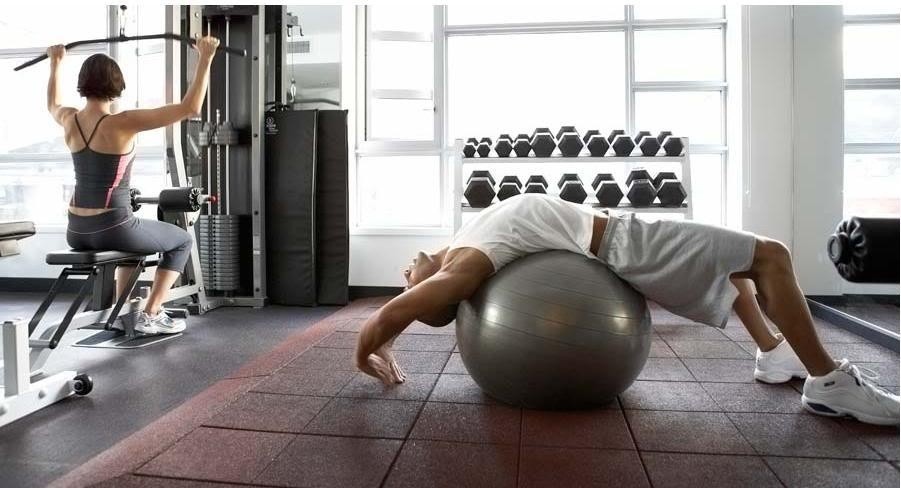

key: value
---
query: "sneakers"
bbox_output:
[132,306,187,335]
[109,315,126,332]
[752,332,811,384]
[800,357,899,429]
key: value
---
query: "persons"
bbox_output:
[45,35,220,336]
[357,192,900,432]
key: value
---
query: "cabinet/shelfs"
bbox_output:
[452,135,694,238]
